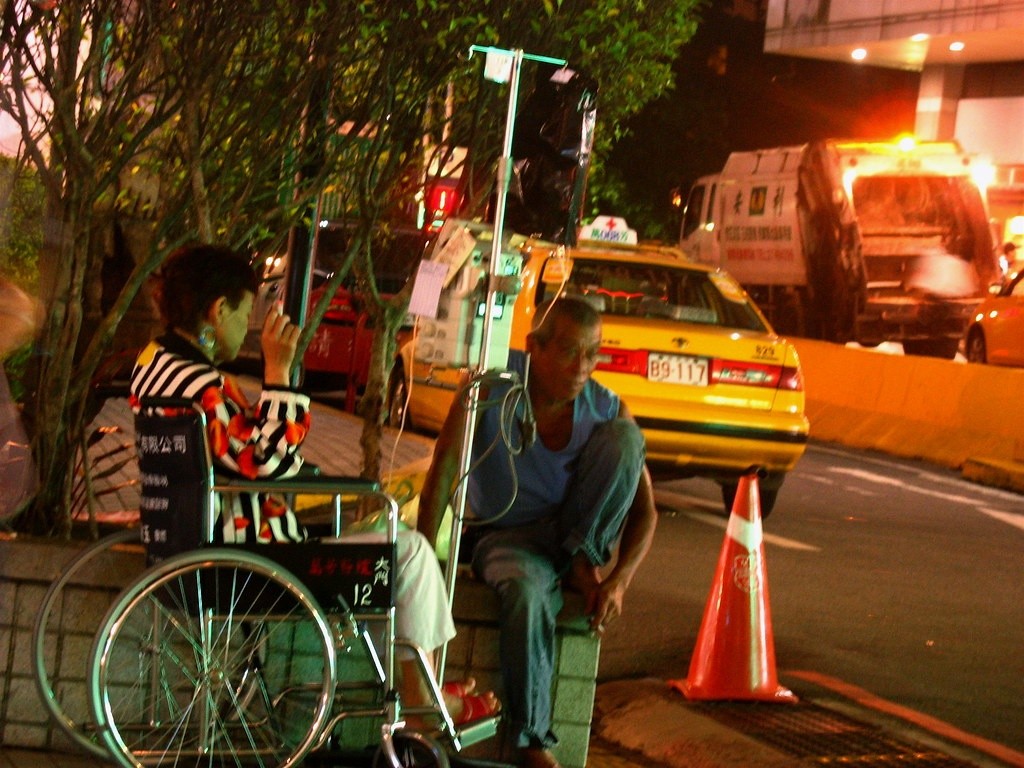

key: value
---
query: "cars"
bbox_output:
[257,253,404,387]
[961,261,1024,366]
[392,216,808,518]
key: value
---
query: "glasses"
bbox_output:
[538,340,595,362]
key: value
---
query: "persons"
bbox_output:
[416,297,658,768]
[130,244,502,739]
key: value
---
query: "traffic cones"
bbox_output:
[669,471,798,704]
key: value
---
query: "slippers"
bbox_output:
[398,677,500,729]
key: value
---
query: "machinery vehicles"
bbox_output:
[686,137,1005,355]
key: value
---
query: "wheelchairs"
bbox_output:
[30,383,505,768]
